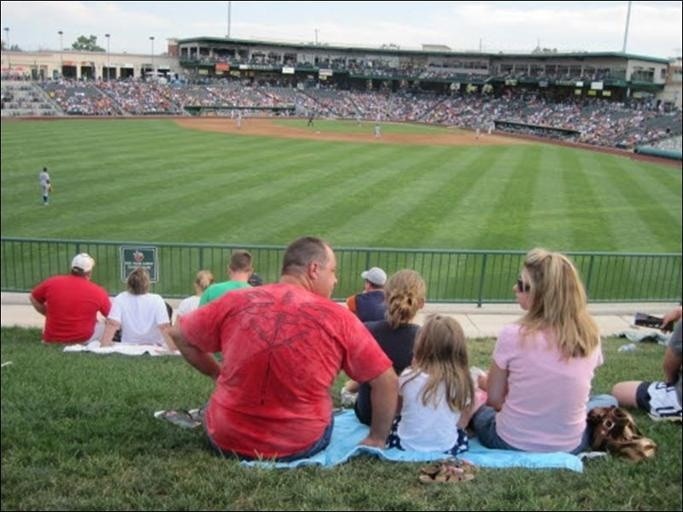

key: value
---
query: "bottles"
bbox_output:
[361,266,387,287]
[71,252,97,275]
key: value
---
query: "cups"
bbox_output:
[517,279,531,294]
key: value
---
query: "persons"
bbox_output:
[30,253,113,346]
[1,52,682,148]
[100,268,177,351]
[612,305,683,420]
[247,274,263,286]
[168,236,399,462]
[386,312,478,455]
[473,248,618,455]
[346,267,390,324]
[171,270,215,326]
[39,167,51,206]
[345,268,427,427]
[198,250,255,363]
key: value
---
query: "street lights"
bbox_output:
[586,404,657,465]
